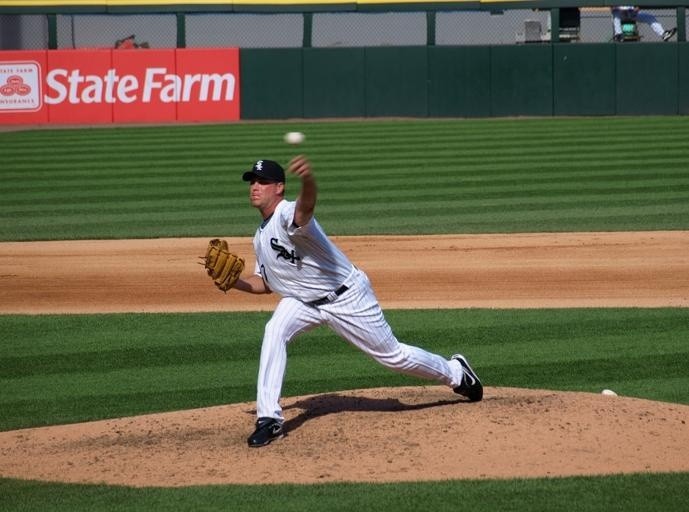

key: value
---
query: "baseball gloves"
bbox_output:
[204,240,245,293]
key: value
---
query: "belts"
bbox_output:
[305,284,348,308]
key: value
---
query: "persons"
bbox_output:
[204,154,483,447]
[611,6,677,42]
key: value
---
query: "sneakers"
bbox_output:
[451,353,485,403]
[247,417,285,447]
[662,27,676,41]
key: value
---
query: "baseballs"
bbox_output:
[286,132,303,144]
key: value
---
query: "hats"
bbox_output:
[241,159,286,184]
